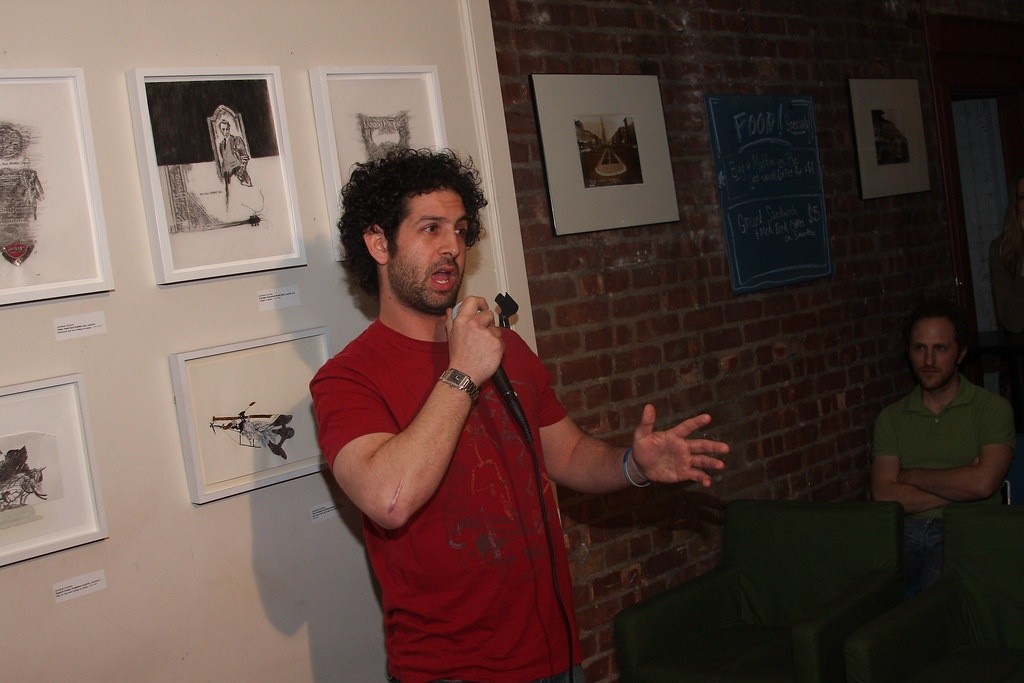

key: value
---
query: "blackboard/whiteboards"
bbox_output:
[703,93,833,294]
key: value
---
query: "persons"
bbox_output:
[989,167,1024,434]
[308,149,730,683]
[871,302,1017,596]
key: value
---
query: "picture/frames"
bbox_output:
[531,72,685,239]
[128,65,308,286]
[0,65,115,306]
[0,372,109,567]
[310,65,448,262]
[845,76,932,202]
[173,325,333,505]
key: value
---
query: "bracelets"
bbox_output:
[623,448,651,487]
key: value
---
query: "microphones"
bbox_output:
[451,301,535,443]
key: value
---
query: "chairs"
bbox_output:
[613,499,1024,683]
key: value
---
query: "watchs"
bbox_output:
[438,368,479,402]
